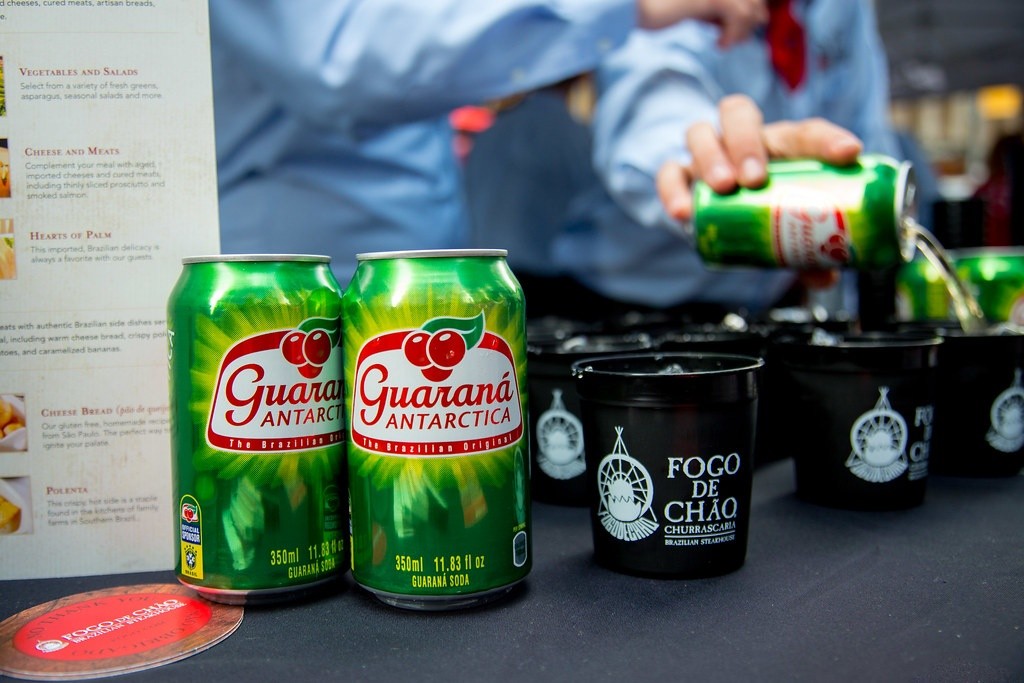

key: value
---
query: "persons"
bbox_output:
[208,0,936,325]
[943,130,1024,248]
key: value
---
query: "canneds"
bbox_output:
[895,246,1024,325]
[689,150,919,268]
[164,248,536,611]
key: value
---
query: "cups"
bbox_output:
[521,304,1024,580]
